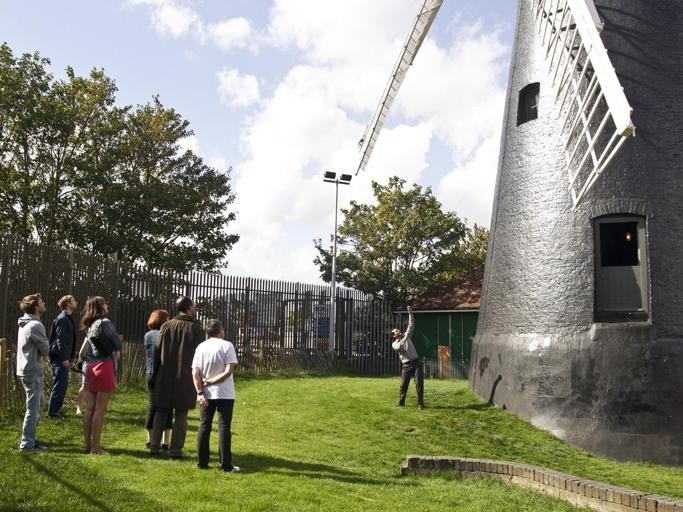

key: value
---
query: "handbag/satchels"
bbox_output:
[88,317,118,358]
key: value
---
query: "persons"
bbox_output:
[390,306,425,409]
[47,295,78,416]
[16,293,50,451]
[191,318,240,472]
[144,310,173,450]
[81,295,123,454]
[76,357,87,416]
[149,297,206,459]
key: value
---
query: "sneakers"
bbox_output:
[144,438,186,459]
[19,439,49,452]
[227,464,241,473]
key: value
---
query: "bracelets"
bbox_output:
[199,392,204,395]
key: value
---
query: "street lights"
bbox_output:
[323,169,352,349]
